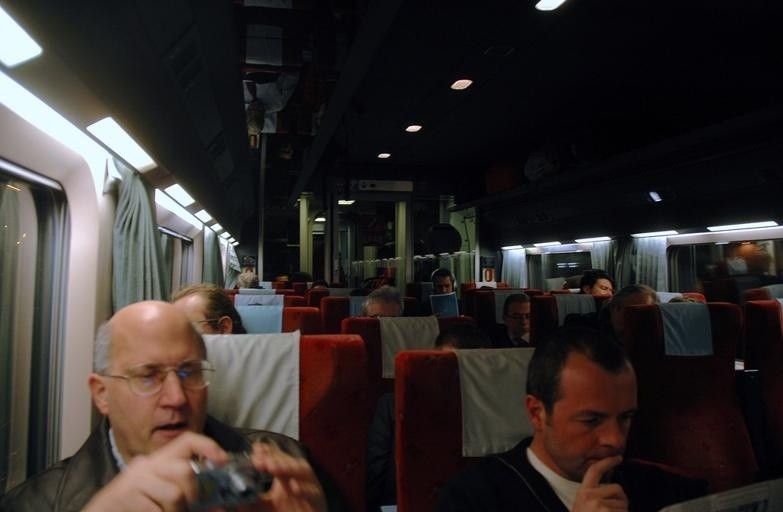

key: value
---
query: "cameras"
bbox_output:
[190,452,273,507]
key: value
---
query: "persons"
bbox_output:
[485,269,660,349]
[362,284,404,319]
[430,268,465,317]
[237,273,264,290]
[434,322,717,512]
[0,300,352,512]
[175,282,244,334]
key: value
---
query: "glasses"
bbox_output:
[502,311,531,323]
[99,356,216,397]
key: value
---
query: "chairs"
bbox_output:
[160,272,781,510]
